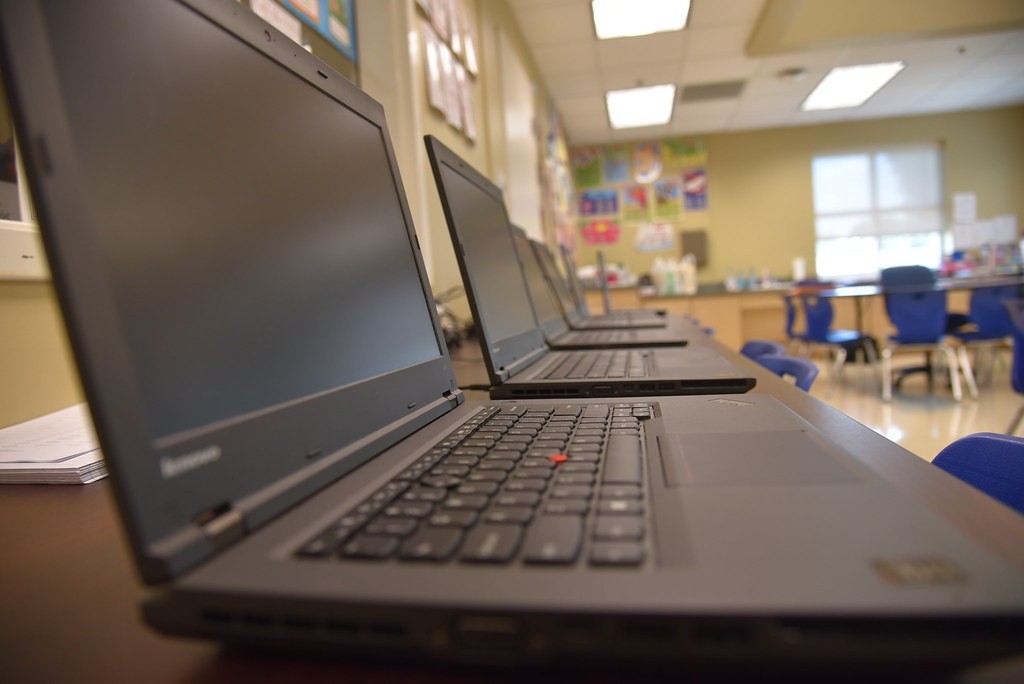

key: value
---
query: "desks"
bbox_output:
[0,275,1024,684]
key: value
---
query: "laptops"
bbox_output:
[0,0,1024,673]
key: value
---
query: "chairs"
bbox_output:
[687,266,1024,516]
